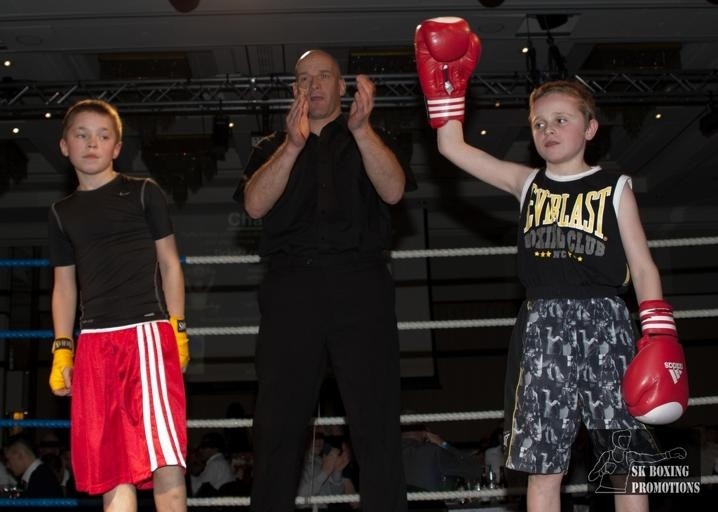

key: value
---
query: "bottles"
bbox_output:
[440,465,497,506]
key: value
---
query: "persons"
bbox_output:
[36,96,193,512]
[416,14,694,512]
[2,418,718,512]
[236,44,418,510]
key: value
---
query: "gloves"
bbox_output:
[167,314,193,371]
[620,299,688,427]
[46,334,76,394]
[413,15,483,129]
